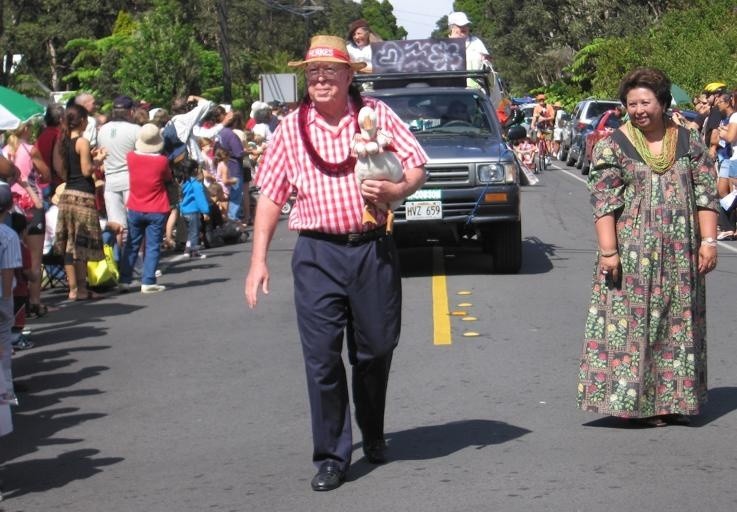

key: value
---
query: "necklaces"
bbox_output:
[465,33,471,48]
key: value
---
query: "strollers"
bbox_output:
[250,187,296,215]
[194,183,254,248]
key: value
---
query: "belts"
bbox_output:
[298,226,387,248]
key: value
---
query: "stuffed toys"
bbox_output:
[349,106,406,237]
[534,109,553,138]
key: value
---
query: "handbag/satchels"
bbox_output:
[87,243,119,284]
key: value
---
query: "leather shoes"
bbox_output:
[310,460,343,491]
[361,431,385,463]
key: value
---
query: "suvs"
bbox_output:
[350,70,525,271]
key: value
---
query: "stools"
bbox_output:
[41,255,69,291]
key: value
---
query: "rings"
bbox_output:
[600,270,610,275]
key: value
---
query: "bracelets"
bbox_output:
[601,249,618,257]
[700,238,717,247]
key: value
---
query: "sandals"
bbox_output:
[716,230,734,240]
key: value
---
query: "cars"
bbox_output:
[515,95,700,186]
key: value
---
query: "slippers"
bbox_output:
[76,292,103,300]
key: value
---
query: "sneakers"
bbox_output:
[141,285,166,294]
[189,253,205,259]
[121,283,129,292]
[11,337,34,351]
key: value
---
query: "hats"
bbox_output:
[552,101,561,108]
[448,11,474,27]
[287,35,367,72]
[135,122,164,153]
[346,20,367,41]
[113,96,133,109]
[537,95,545,100]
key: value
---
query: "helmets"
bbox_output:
[703,81,727,95]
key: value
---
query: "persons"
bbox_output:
[613,104,626,126]
[448,11,493,98]
[576,65,721,426]
[672,82,736,240]
[531,93,555,166]
[445,100,467,126]
[245,35,430,490]
[1,85,295,314]
[0,222,22,435]
[347,18,372,75]
